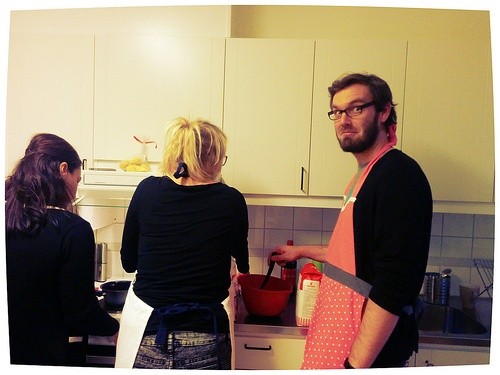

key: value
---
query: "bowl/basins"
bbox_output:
[237,274,293,316]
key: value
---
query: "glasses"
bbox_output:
[219,155,228,166]
[327,101,376,121]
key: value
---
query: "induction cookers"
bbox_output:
[88,298,122,344]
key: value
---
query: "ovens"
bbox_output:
[86,344,116,368]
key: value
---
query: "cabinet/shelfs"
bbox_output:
[5,4,232,171]
[234,335,307,370]
[308,39,495,215]
[220,37,316,207]
[415,342,490,368]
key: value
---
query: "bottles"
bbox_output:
[280,240,298,298]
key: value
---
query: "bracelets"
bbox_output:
[343,358,355,369]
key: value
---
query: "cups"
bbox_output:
[459,284,480,309]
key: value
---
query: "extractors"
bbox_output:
[78,169,161,186]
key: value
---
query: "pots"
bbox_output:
[94,281,132,311]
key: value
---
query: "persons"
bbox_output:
[5,133,120,368]
[120,117,250,370]
[267,73,434,368]
